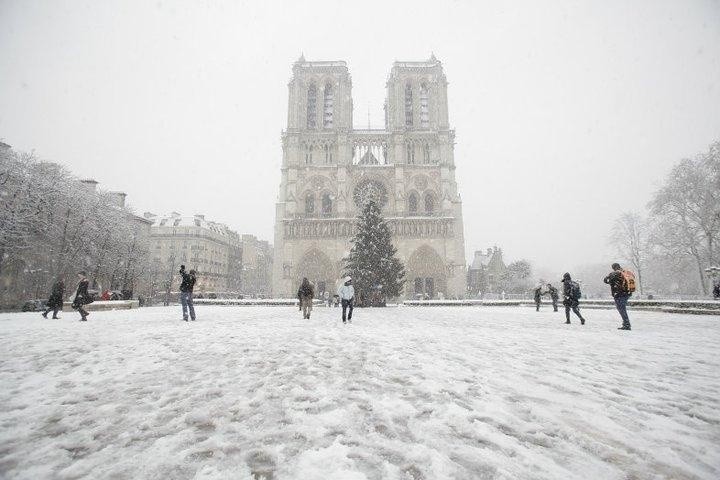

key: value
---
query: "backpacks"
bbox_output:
[621,270,636,292]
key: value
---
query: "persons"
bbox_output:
[561,272,586,325]
[603,262,636,330]
[42,277,65,319]
[297,277,315,319]
[534,284,559,312]
[71,270,94,321]
[179,264,197,321]
[337,276,355,324]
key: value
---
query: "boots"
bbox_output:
[42,313,60,319]
[80,310,89,321]
[564,318,585,324]
[343,319,352,325]
[183,316,196,321]
[618,321,631,330]
[302,315,311,320]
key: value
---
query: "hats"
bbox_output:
[561,272,571,282]
[344,276,352,283]
